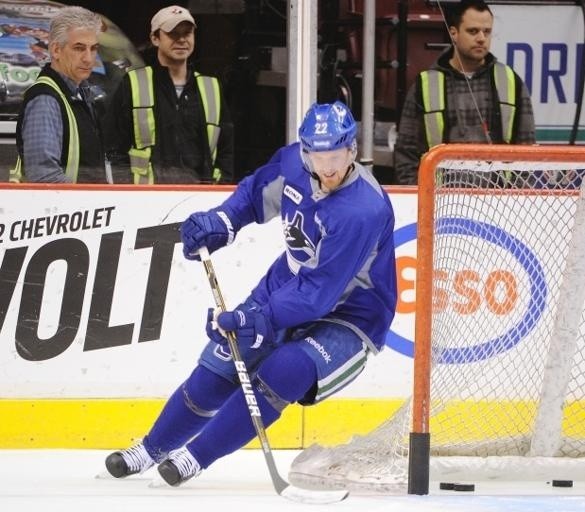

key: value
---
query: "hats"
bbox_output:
[149,4,198,35]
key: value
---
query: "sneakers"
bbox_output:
[105,441,156,480]
[157,446,204,487]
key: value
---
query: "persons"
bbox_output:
[393,0,536,189]
[8,6,107,184]
[105,101,397,486]
[101,6,235,184]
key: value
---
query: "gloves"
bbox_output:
[204,300,279,350]
[177,203,238,262]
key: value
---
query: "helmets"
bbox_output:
[297,101,358,173]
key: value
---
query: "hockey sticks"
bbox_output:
[199,245,350,503]
